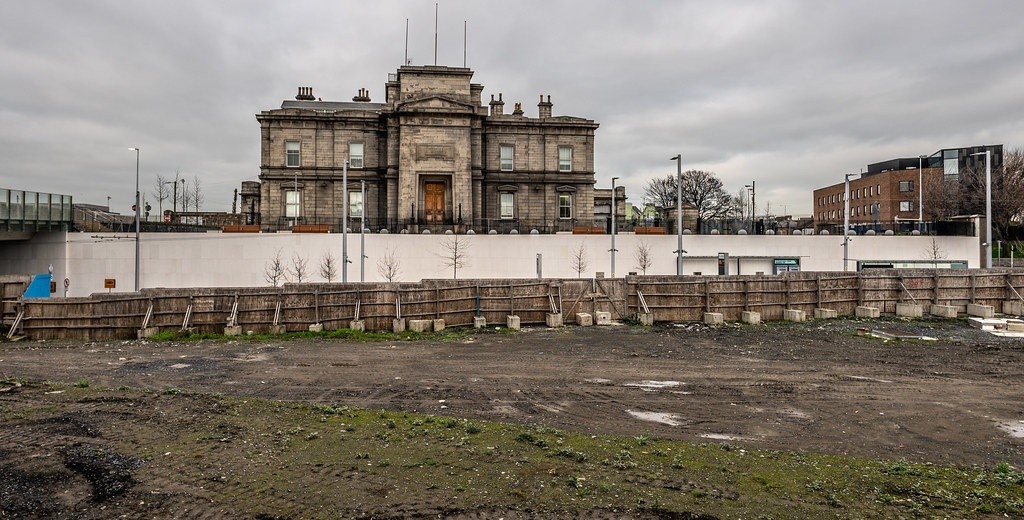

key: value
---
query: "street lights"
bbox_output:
[342,159,350,282]
[918,154,927,222]
[611,176,620,278]
[127,147,140,292]
[294,171,303,225]
[361,180,366,281]
[969,150,993,269]
[669,153,684,275]
[843,173,857,270]
[744,180,756,236]
